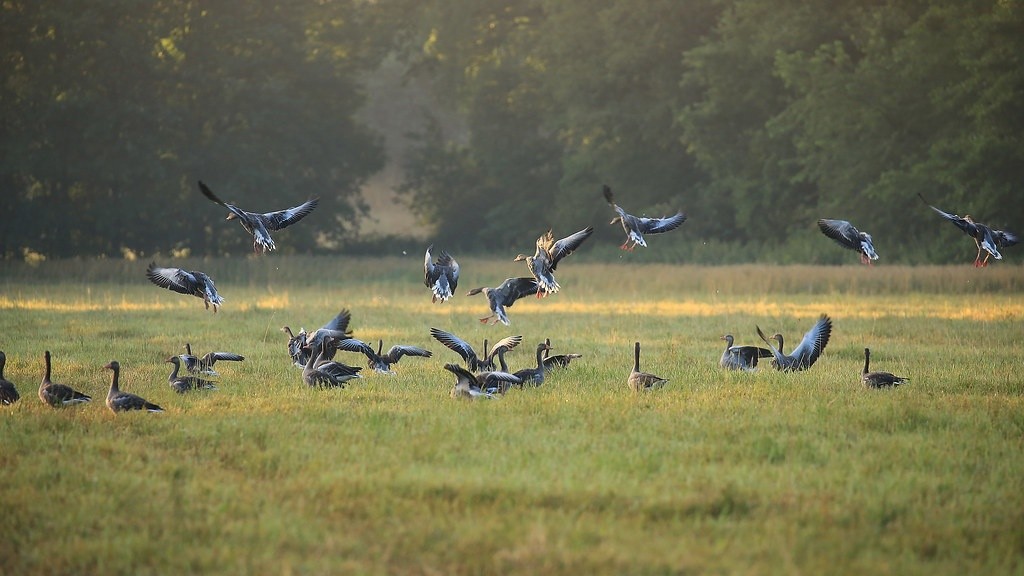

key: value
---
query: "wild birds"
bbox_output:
[466,277,553,326]
[280,307,584,401]
[860,347,910,390]
[719,335,774,372]
[424,244,460,303]
[514,227,594,297]
[38,350,92,407]
[164,356,218,394]
[917,192,1020,269]
[179,343,246,377]
[756,313,833,374]
[0,350,21,404]
[817,219,879,271]
[103,361,166,413]
[197,179,323,254]
[601,184,687,252]
[627,342,670,392]
[145,260,226,314]
